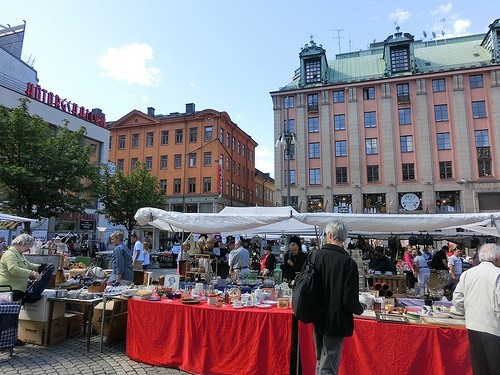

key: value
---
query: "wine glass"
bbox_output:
[106,286,129,295]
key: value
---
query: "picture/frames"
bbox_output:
[163,274,179,292]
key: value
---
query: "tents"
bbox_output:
[134,206,500,275]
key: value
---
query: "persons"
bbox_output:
[0,234,42,346]
[107,231,134,286]
[299,220,367,375]
[131,233,144,270]
[177,236,479,296]
[0,236,7,253]
[142,242,150,269]
[453,243,500,375]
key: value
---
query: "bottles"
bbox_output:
[274,264,283,286]
[278,287,283,298]
[209,267,274,307]
[397,265,403,275]
[277,298,289,309]
[425,293,433,312]
[198,265,204,273]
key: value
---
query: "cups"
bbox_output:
[375,271,380,273]
[386,272,391,274]
[422,306,432,315]
[396,307,407,314]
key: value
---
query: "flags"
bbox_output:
[440,16,448,22]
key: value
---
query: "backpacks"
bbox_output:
[292,250,321,324]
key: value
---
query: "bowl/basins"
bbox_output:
[190,268,198,272]
[55,290,99,300]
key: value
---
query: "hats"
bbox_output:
[289,236,300,246]
[207,238,217,243]
[262,244,272,251]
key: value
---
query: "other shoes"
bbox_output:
[16,339,26,346]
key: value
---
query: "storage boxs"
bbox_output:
[18,268,115,346]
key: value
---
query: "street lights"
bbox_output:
[275,108,300,207]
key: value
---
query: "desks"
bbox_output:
[45,290,473,375]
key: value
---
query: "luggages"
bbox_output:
[0,285,21,357]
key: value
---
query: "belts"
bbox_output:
[418,266,429,269]
[235,266,247,269]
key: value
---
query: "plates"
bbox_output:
[233,306,243,308]
[181,300,200,303]
[374,273,381,275]
[385,274,392,276]
[258,305,272,308]
[264,302,278,304]
[243,305,254,308]
